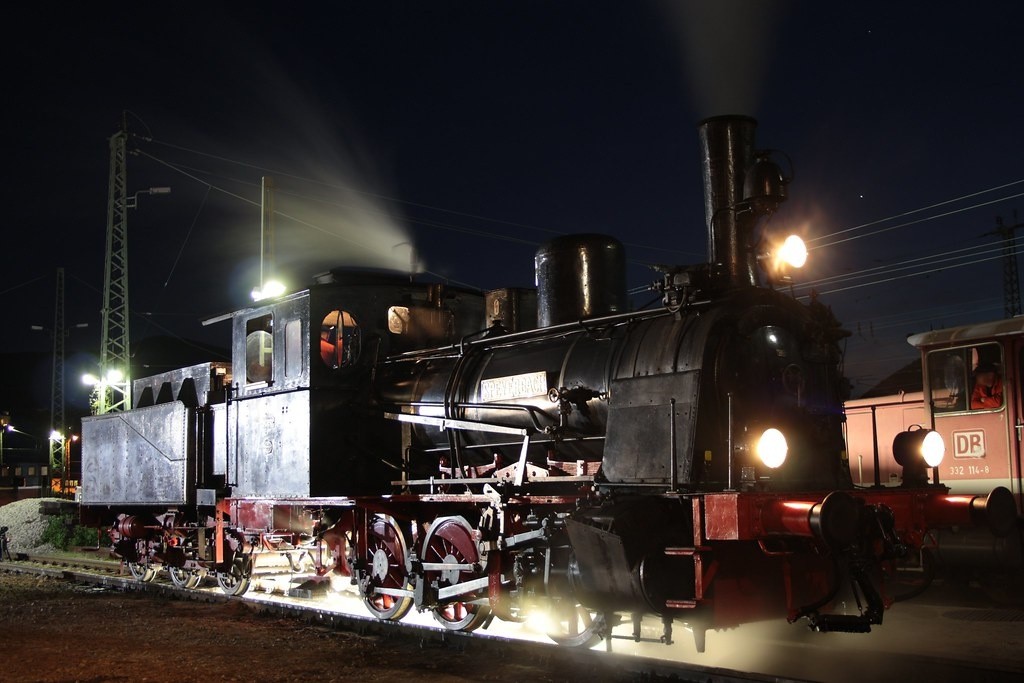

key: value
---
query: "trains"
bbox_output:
[74,114,1024,655]
[840,316,1024,577]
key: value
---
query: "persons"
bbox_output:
[970,362,1003,411]
[317,325,335,367]
